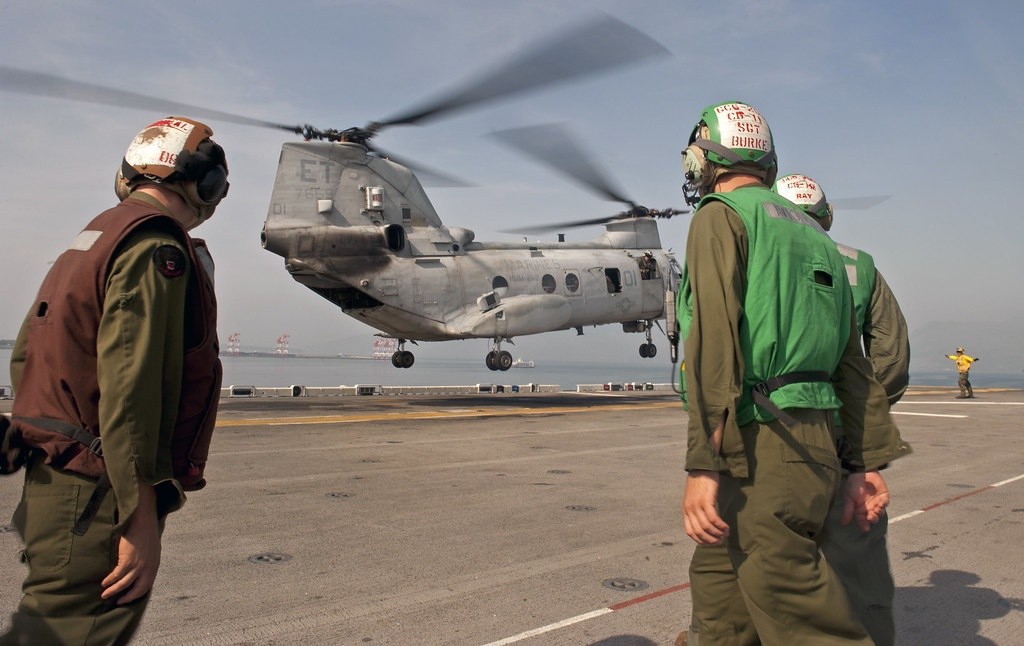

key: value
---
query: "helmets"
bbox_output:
[695,101,778,201]
[771,173,832,231]
[115,117,229,211]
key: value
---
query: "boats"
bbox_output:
[512,356,536,369]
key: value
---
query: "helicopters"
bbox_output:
[0,7,693,371]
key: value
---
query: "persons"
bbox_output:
[676,99,913,645]
[2,116,230,646]
[945,347,980,399]
[639,250,657,280]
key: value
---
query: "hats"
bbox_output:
[956,347,964,353]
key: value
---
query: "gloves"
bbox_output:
[974,358,979,361]
